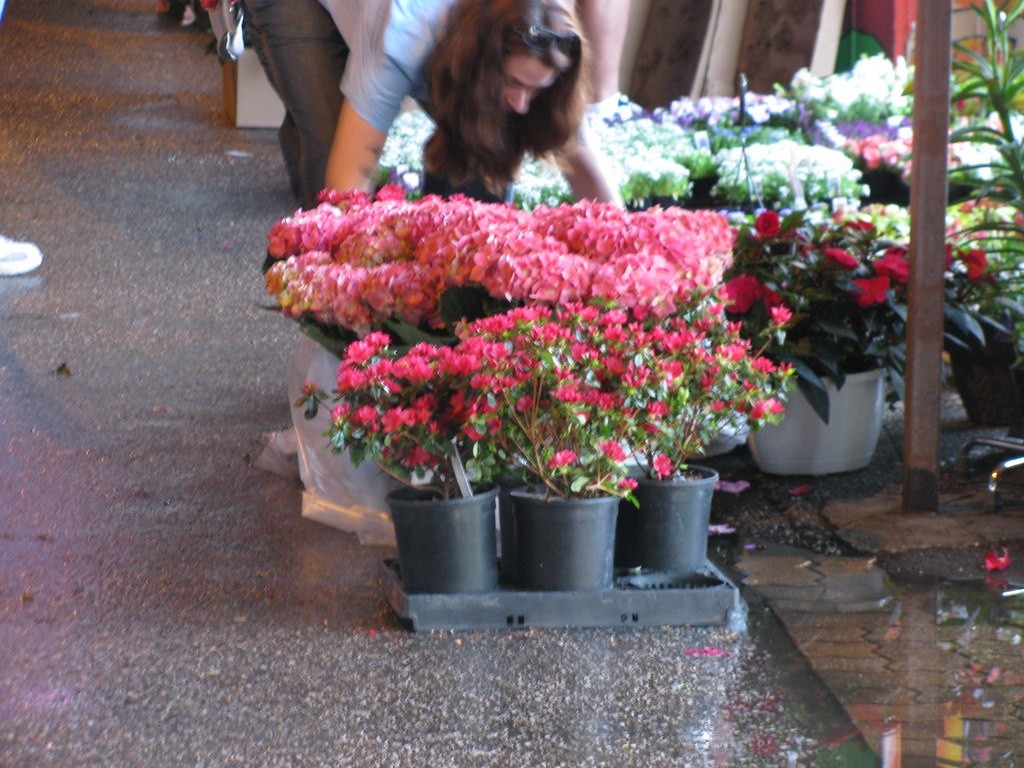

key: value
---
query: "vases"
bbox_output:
[619,462,720,569]
[743,368,888,474]
[950,344,1018,428]
[383,479,504,593]
[510,482,623,589]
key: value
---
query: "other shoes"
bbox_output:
[182,11,211,30]
[159,6,186,21]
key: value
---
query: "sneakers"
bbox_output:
[0,235,43,277]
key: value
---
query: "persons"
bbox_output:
[244,0,627,212]
[0,230,45,279]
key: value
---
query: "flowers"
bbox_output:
[262,51,1024,508]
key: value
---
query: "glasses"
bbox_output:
[503,25,580,57]
